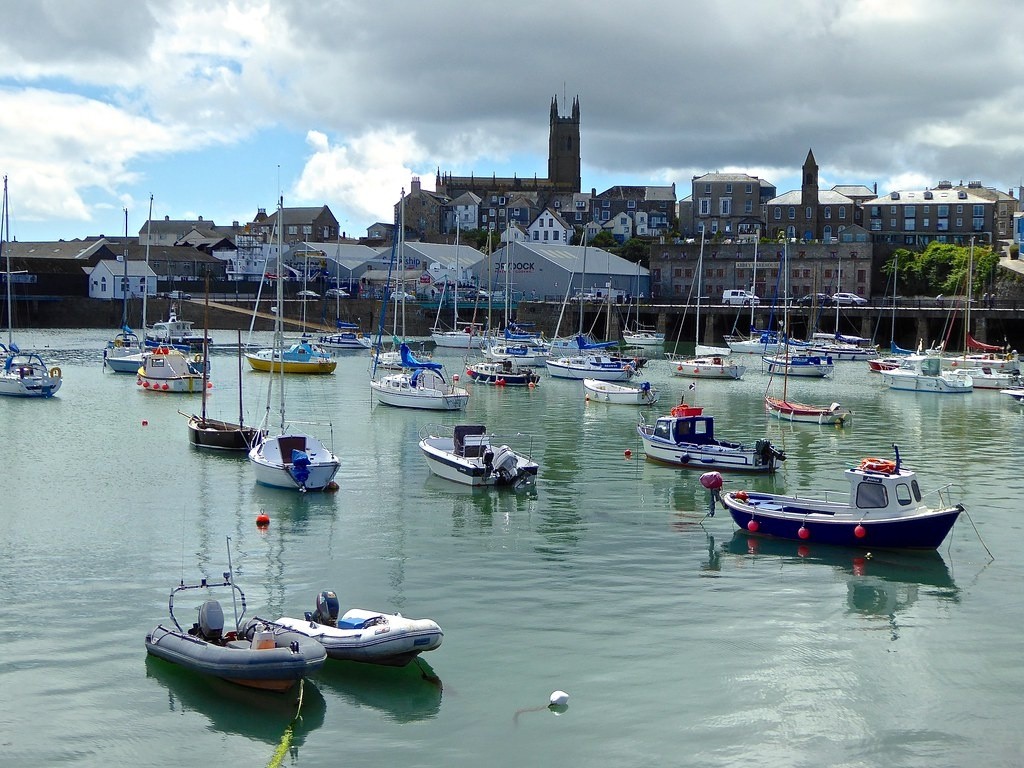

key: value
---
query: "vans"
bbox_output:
[722,290,760,305]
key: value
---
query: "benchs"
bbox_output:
[759,503,787,511]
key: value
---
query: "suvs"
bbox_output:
[390,291,416,302]
[832,292,867,306]
[324,289,350,299]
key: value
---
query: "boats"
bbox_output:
[419,422,539,489]
[700,443,968,554]
[637,396,786,473]
[272,590,444,668]
[145,535,329,693]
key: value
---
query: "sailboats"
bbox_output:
[188,267,270,451]
[0,188,1024,423]
[242,195,340,492]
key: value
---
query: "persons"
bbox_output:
[935,293,945,307]
[650,292,655,304]
[501,290,506,301]
[602,293,609,303]
[521,290,525,301]
[639,292,644,297]
[991,294,996,308]
[983,293,989,307]
[532,289,535,301]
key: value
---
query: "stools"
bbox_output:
[338,618,365,630]
[228,640,251,649]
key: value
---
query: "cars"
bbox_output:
[164,290,192,300]
[796,292,837,306]
[465,289,489,300]
[296,291,321,300]
[570,292,603,303]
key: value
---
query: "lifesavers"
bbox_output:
[50,367,61,378]
[671,404,689,417]
[1006,353,1012,361]
[114,340,122,347]
[862,457,895,473]
[194,354,201,364]
[357,332,363,339]
